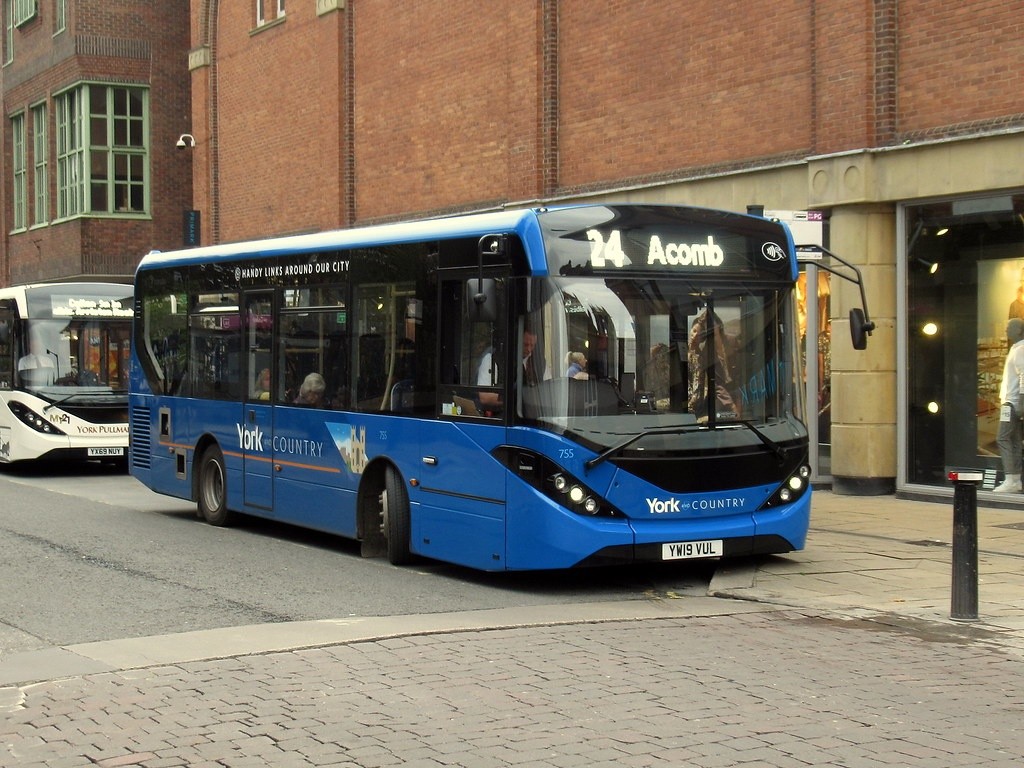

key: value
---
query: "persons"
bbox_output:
[18,335,55,386]
[478,324,589,419]
[650,309,745,417]
[993,318,1024,493]
[255,367,330,408]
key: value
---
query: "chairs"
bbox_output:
[76,370,97,386]
[253,329,420,412]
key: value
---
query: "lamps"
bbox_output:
[936,221,949,235]
[916,257,938,274]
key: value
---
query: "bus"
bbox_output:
[124,202,876,583]
[0,281,135,469]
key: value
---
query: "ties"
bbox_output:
[33,356,41,368]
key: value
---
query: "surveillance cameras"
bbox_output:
[177,141,186,150]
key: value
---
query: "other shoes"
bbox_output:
[1015,480,1022,492]
[993,481,1015,492]
